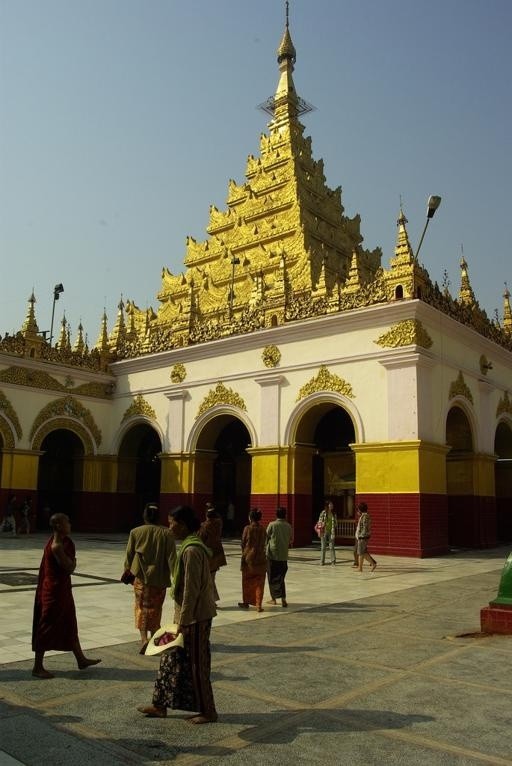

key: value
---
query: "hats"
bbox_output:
[145,623,184,656]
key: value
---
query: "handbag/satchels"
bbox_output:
[315,522,325,536]
[121,570,136,585]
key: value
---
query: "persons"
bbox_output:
[196,501,227,608]
[237,507,268,612]
[353,502,377,572]
[266,507,292,607]
[31,512,102,679]
[123,502,177,654]
[134,505,218,725]
[316,499,339,566]
[351,503,373,568]
[0,493,34,538]
